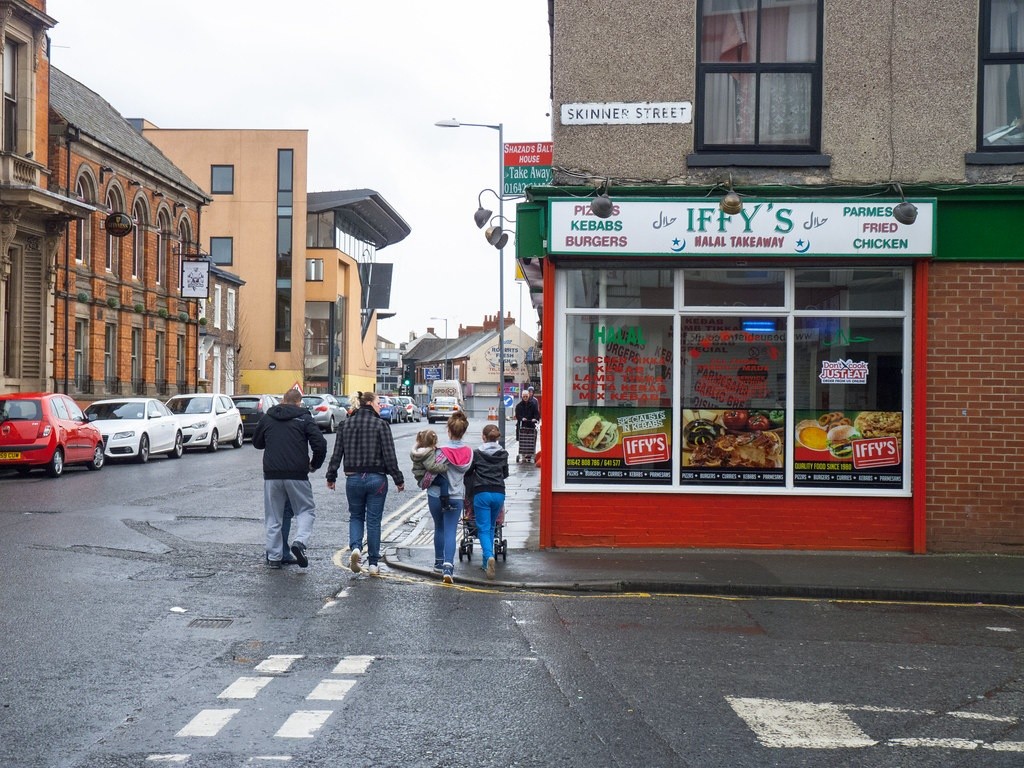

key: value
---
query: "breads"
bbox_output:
[683,409,718,422]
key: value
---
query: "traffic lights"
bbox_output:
[404,370,411,386]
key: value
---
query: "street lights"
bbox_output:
[434,118,507,450]
[515,282,523,402]
[429,316,447,381]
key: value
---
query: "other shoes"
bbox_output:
[485,557,496,580]
[284,556,298,564]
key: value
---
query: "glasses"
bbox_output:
[523,396,528,399]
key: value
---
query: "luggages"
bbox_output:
[516,419,538,462]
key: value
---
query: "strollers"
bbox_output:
[457,498,508,562]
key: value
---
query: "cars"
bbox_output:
[83,398,185,464]
[0,392,106,479]
[273,395,315,424]
[335,394,353,417]
[377,395,429,425]
[302,392,350,434]
[425,396,464,424]
[228,393,280,439]
[161,392,244,453]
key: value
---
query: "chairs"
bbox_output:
[8,406,22,418]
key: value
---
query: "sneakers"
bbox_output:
[442,567,454,584]
[350,547,361,573]
[291,540,308,568]
[434,562,443,572]
[369,565,380,575]
[269,561,281,568]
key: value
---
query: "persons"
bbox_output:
[305,329,314,355]
[515,390,541,464]
[421,412,473,584]
[528,385,539,412]
[253,389,328,569]
[464,425,508,577]
[410,430,458,510]
[326,391,404,575]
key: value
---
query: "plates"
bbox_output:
[576,427,619,453]
[794,419,831,453]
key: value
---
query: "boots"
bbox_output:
[439,494,457,513]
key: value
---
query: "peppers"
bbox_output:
[722,410,784,431]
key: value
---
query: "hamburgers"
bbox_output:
[825,425,864,458]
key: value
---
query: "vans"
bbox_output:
[429,379,467,408]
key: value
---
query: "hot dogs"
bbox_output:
[577,416,611,449]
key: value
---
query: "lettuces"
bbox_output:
[568,411,607,445]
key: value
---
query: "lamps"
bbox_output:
[174,202,185,215]
[890,179,917,224]
[721,171,742,214]
[590,175,614,218]
[128,180,140,189]
[100,166,112,183]
[152,191,163,201]
[474,189,529,248]
